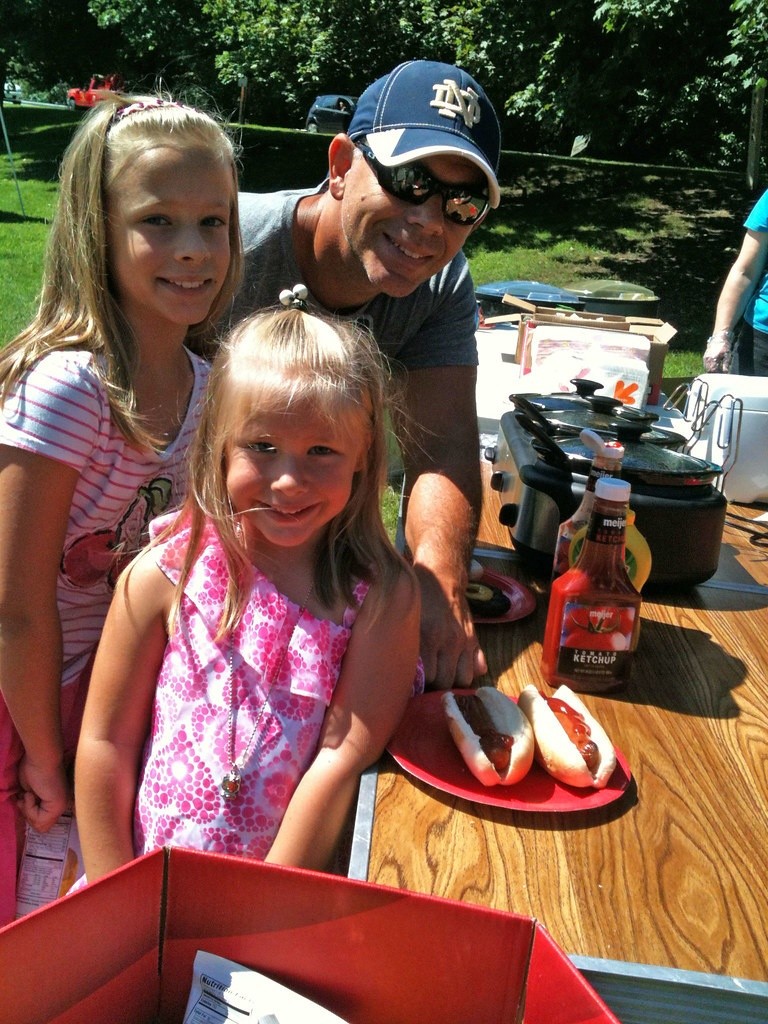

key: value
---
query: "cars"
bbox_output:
[305,94,361,134]
[2,82,23,105]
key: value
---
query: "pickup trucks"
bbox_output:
[65,72,125,110]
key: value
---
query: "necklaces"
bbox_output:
[222,520,317,797]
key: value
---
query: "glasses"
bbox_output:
[354,141,490,225]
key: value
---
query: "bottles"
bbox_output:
[539,478,652,693]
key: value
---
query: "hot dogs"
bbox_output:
[517,684,618,790]
[442,686,534,786]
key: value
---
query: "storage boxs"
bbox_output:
[0,845,623,1023]
[483,293,678,411]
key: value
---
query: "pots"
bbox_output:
[510,376,725,498]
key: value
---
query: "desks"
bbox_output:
[349,456,768,1024]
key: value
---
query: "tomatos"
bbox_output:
[563,605,635,650]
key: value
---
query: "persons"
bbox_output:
[0,93,241,925]
[60,284,423,900]
[702,187,768,377]
[190,59,501,692]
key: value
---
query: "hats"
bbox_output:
[346,60,501,210]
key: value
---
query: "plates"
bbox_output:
[466,567,537,624]
[387,688,630,811]
